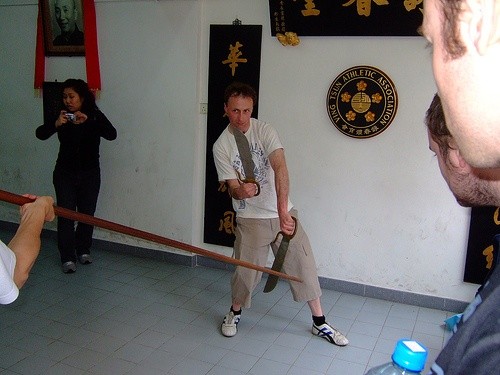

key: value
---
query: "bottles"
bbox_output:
[365,338,429,375]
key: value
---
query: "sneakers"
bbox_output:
[80,253,92,265]
[221,311,241,337]
[63,260,76,272]
[312,322,348,345]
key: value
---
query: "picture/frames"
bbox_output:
[43,0,85,57]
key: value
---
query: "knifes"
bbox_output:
[263,215,298,293]
[231,126,260,197]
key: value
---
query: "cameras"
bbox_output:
[64,114,76,122]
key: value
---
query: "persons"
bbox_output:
[35,78,117,273]
[416,0,500,375]
[0,193,55,304]
[212,83,350,346]
[52,0,86,46]
